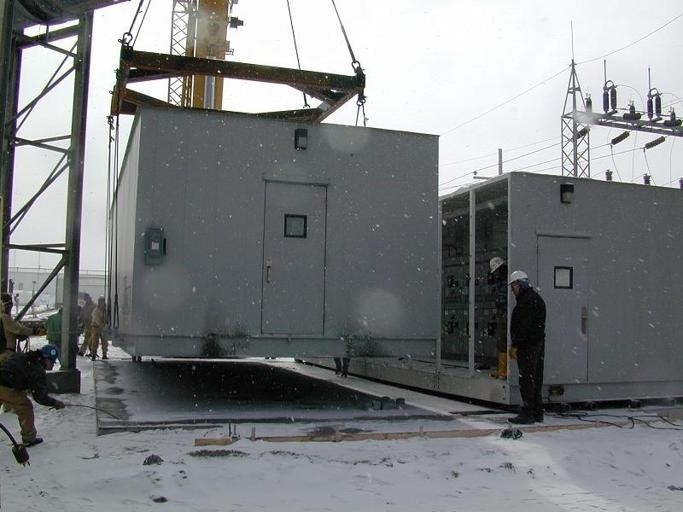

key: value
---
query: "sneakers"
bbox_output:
[78,352,107,361]
[508,413,543,424]
[23,438,42,446]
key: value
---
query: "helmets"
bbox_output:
[40,345,57,360]
[508,271,528,285]
[490,257,504,273]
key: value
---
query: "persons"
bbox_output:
[13,292,19,316]
[506,270,548,426]
[44,292,108,367]
[0,345,65,449]
[332,356,352,378]
[485,256,509,382]
[0,291,46,416]
